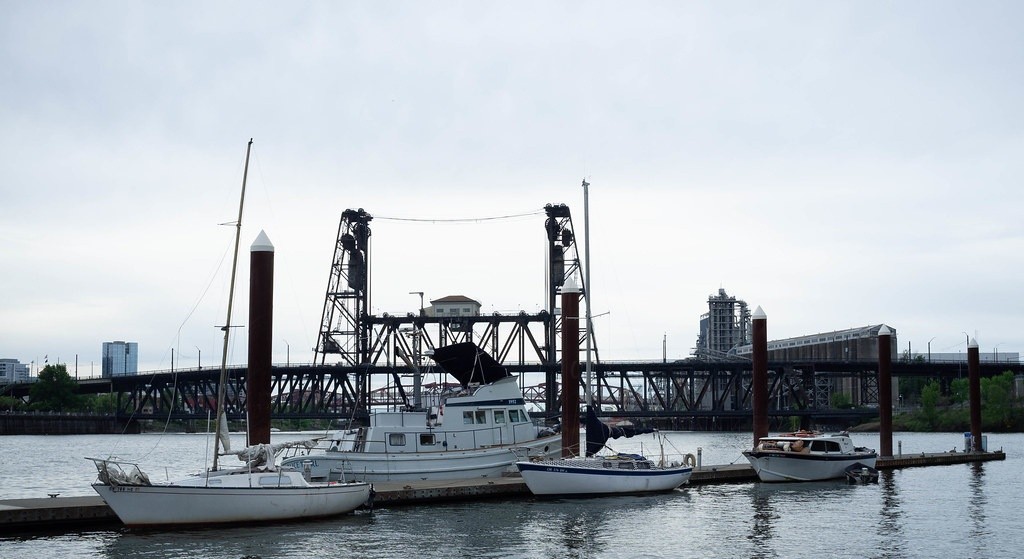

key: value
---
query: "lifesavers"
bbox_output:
[683,452,696,469]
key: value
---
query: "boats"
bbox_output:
[843,461,880,487]
[739,429,880,484]
[280,202,588,502]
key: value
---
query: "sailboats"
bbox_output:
[83,136,377,532]
[515,178,696,500]
